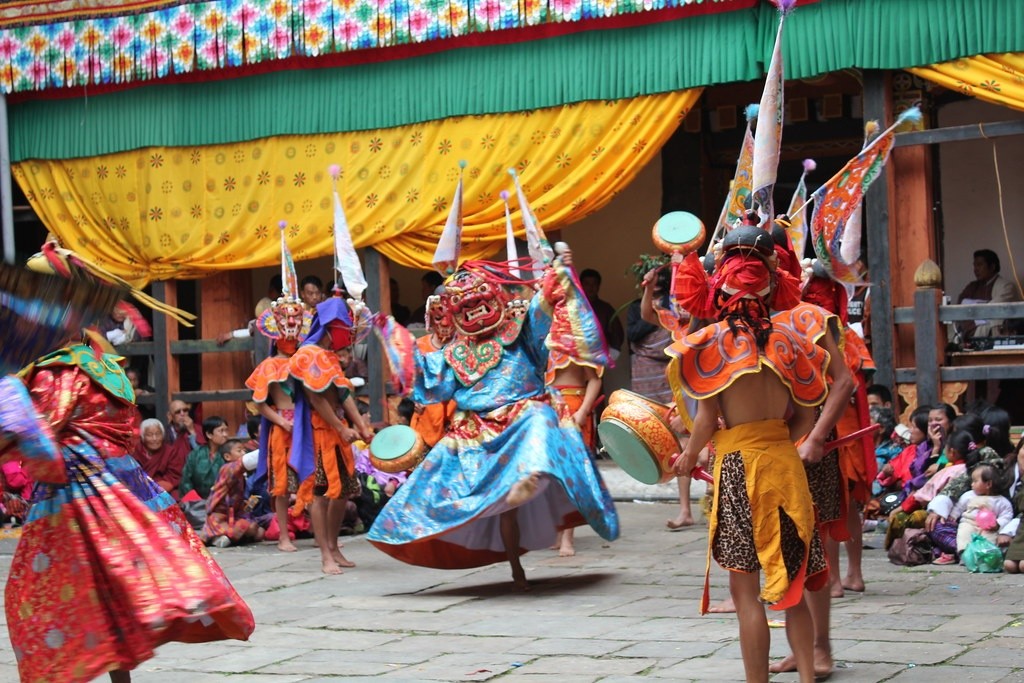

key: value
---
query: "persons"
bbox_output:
[366,248,618,594]
[953,250,1020,347]
[246,192,370,573]
[0,240,256,683]
[98,299,151,386]
[167,398,207,464]
[414,160,466,452]
[179,416,229,530]
[414,272,444,324]
[216,273,282,367]
[888,403,1024,571]
[326,277,411,326]
[198,438,266,548]
[300,275,324,305]
[132,418,186,503]
[345,398,412,531]
[619,0,922,683]
[500,167,624,557]
[124,366,156,419]
[397,398,431,479]
[993,317,1024,426]
[244,415,279,539]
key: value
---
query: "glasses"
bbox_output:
[174,407,190,415]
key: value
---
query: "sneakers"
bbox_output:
[933,553,955,564]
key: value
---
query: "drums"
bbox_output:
[368,424,424,474]
[597,398,683,485]
[652,211,706,255]
[608,388,675,427]
